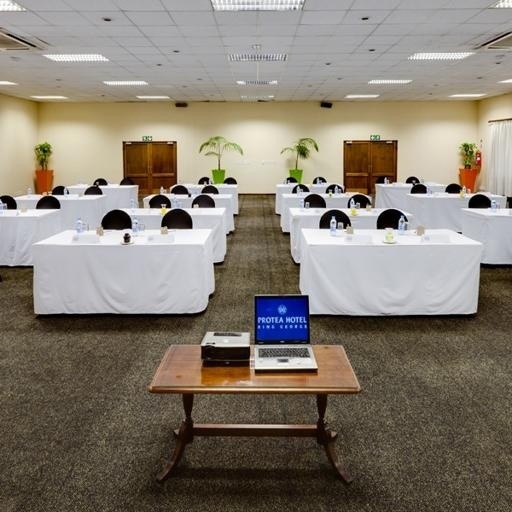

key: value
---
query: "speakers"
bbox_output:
[320,102,332,108]
[175,103,188,107]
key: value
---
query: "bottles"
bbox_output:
[329,216,337,236]
[334,185,338,195]
[300,198,305,212]
[329,189,332,198]
[75,216,83,233]
[123,233,131,244]
[350,198,355,209]
[317,177,320,185]
[385,228,394,242]
[490,200,498,212]
[460,190,465,198]
[297,185,300,196]
[27,187,33,199]
[131,216,139,234]
[130,196,135,212]
[63,187,68,199]
[351,205,357,216]
[463,185,467,195]
[161,203,167,215]
[398,214,405,234]
[384,177,387,184]
[283,175,287,184]
[172,196,179,208]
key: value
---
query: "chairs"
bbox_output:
[0,175,241,316]
[273,173,512,322]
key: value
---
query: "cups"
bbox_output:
[287,179,290,184]
[138,223,146,233]
[299,189,303,195]
[81,221,89,232]
[305,202,310,211]
[337,221,345,237]
[355,202,361,209]
[404,221,411,235]
[338,189,342,194]
[387,180,390,184]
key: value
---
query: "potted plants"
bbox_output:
[35,142,54,195]
[281,136,321,183]
[198,136,244,183]
[458,142,478,192]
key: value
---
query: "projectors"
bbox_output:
[201,331,250,363]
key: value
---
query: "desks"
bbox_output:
[147,343,363,486]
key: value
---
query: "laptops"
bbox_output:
[254,295,318,372]
